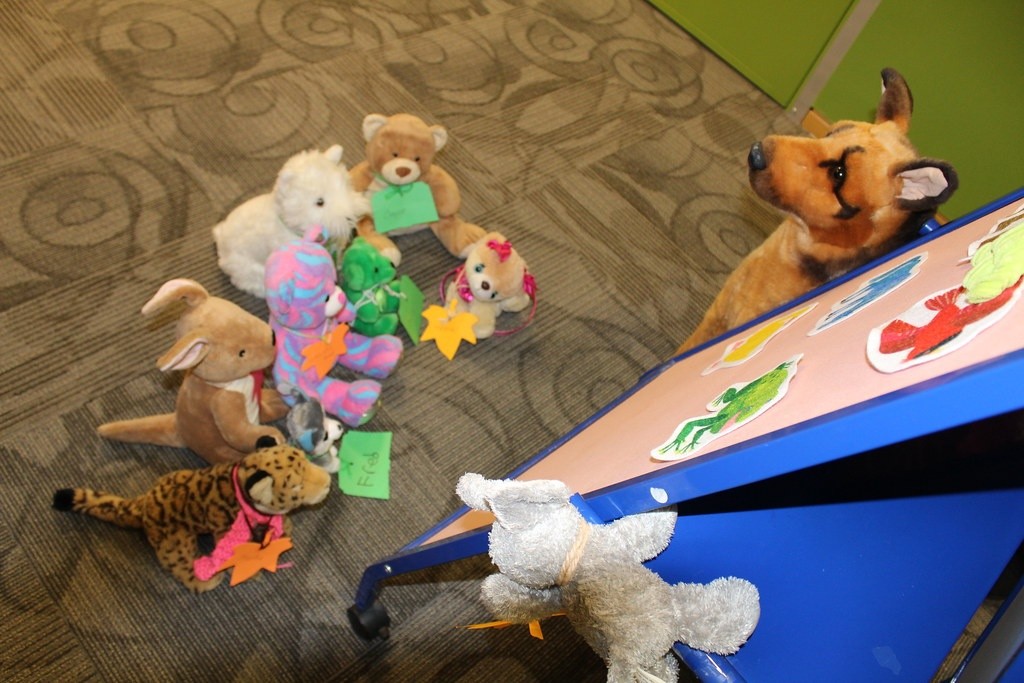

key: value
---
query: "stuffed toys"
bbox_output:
[455,472,760,683]
[213,113,538,427]
[674,65,960,357]
[53,278,344,594]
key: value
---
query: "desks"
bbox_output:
[604,412,1024,683]
